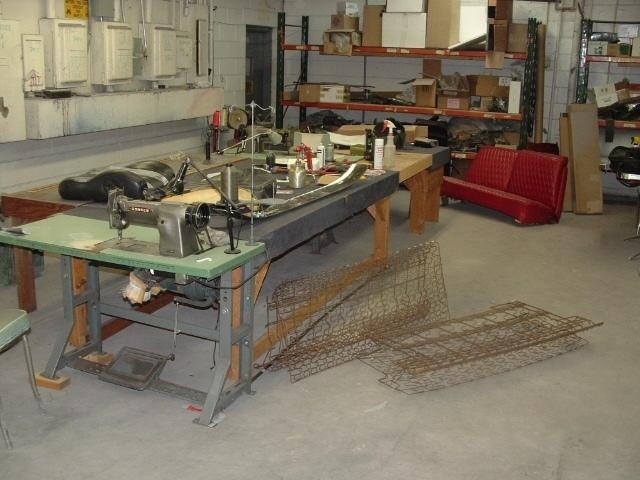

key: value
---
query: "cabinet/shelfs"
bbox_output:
[576,18,640,142]
[275,11,542,207]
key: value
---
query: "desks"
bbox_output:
[0,136,452,381]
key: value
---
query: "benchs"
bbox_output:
[439,146,569,227]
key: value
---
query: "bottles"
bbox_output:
[287,164,306,188]
[325,143,335,162]
[221,162,239,206]
[212,107,228,128]
[365,126,384,170]
